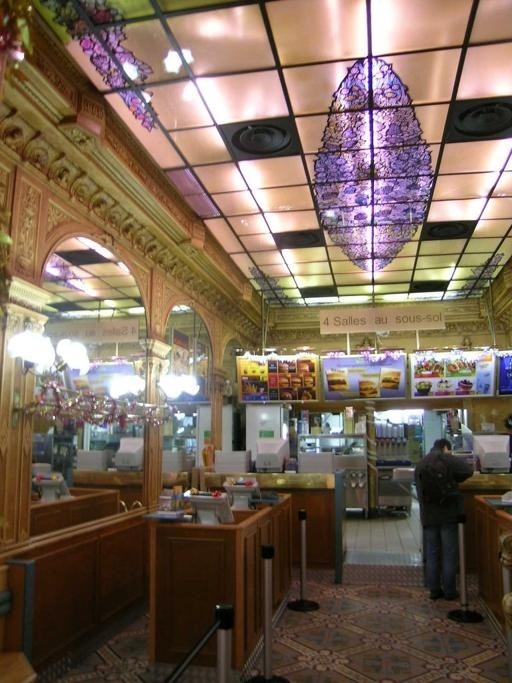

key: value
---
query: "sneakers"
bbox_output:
[445,590,459,601]
[430,588,444,600]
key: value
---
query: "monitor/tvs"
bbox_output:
[115,437,144,473]
[472,434,511,473]
[256,437,290,473]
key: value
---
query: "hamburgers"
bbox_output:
[292,378,302,388]
[359,380,379,397]
[327,372,349,390]
[305,378,313,388]
[382,375,400,389]
[281,378,290,388]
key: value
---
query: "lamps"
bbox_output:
[48,338,89,378]
[8,329,56,376]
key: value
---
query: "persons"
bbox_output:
[415,438,473,601]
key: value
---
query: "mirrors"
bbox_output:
[24,234,248,537]
